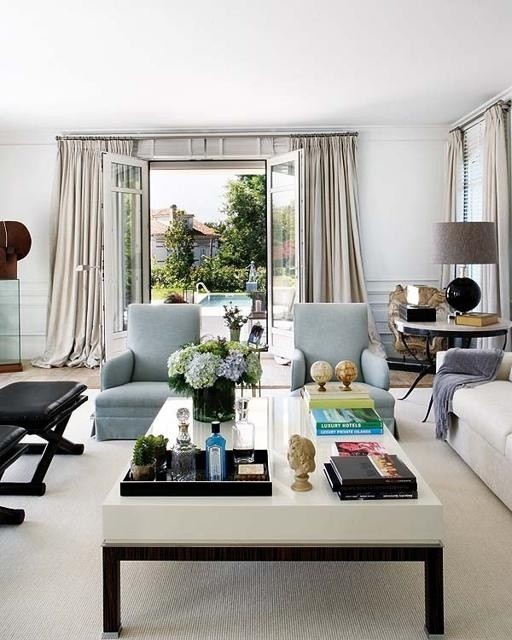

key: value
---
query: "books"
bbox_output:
[302,383,384,435]
[323,442,418,500]
[455,313,499,327]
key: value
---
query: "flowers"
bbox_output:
[164,332,265,422]
[220,299,250,330]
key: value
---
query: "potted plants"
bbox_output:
[129,437,157,480]
[147,434,168,469]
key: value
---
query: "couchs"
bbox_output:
[430,349,511,516]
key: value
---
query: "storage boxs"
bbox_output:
[398,303,437,323]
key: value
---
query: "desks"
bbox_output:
[392,315,512,423]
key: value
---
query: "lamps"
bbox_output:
[430,219,500,321]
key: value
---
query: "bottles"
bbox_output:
[170,408,197,481]
[255,299,261,311]
[205,420,228,481]
[231,397,255,466]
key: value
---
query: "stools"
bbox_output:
[0,381,89,495]
[0,424,31,527]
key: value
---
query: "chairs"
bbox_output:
[386,281,453,366]
[289,301,399,440]
[92,303,204,442]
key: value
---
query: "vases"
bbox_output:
[191,375,237,425]
[230,330,241,342]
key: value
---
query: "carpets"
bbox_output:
[2,389,512,639]
